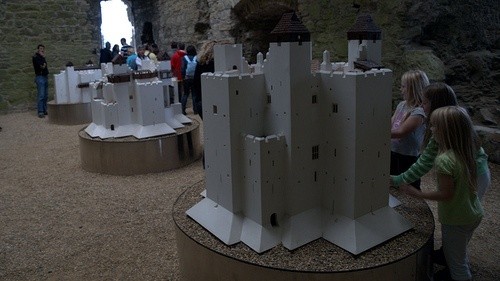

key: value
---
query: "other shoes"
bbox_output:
[38,112,45,118]
[45,112,49,115]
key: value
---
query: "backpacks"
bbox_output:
[184,55,198,81]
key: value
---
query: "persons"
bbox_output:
[191,37,234,169]
[98,38,171,71]
[167,41,198,115]
[399,105,485,281]
[390,70,430,191]
[32,44,49,118]
[389,83,490,265]
